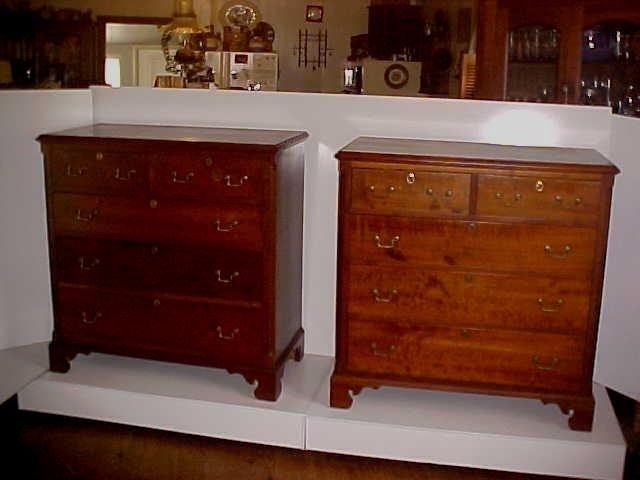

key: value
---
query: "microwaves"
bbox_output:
[344,62,422,96]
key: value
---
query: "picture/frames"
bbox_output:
[305,5,324,23]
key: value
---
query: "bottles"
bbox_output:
[513,28,558,60]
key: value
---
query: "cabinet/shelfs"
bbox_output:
[471,0,640,119]
[34,123,309,403]
[330,136,621,433]
